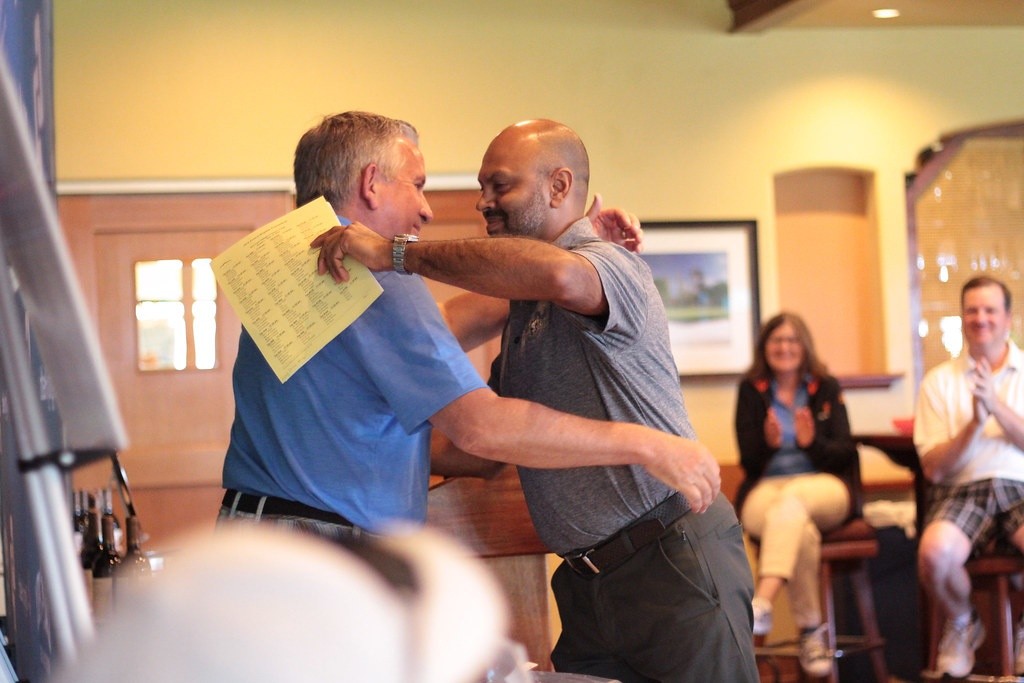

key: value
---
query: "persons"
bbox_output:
[913,276,1024,679]
[215,111,723,552]
[734,312,868,678]
[311,117,761,683]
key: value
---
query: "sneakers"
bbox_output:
[1015,615,1024,677]
[751,596,774,634]
[936,612,986,678]
[800,623,831,677]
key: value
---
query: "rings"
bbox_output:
[622,230,626,239]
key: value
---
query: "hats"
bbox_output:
[79,522,510,683]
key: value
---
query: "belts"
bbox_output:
[222,490,353,527]
[562,490,691,579]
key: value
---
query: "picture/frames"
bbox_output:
[640,220,763,384]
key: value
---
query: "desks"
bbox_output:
[851,435,928,546]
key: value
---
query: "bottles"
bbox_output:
[72,487,151,628]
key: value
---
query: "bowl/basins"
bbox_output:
[892,417,913,438]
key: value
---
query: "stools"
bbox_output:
[749,517,893,683]
[920,536,1024,683]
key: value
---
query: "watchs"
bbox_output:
[392,234,420,275]
